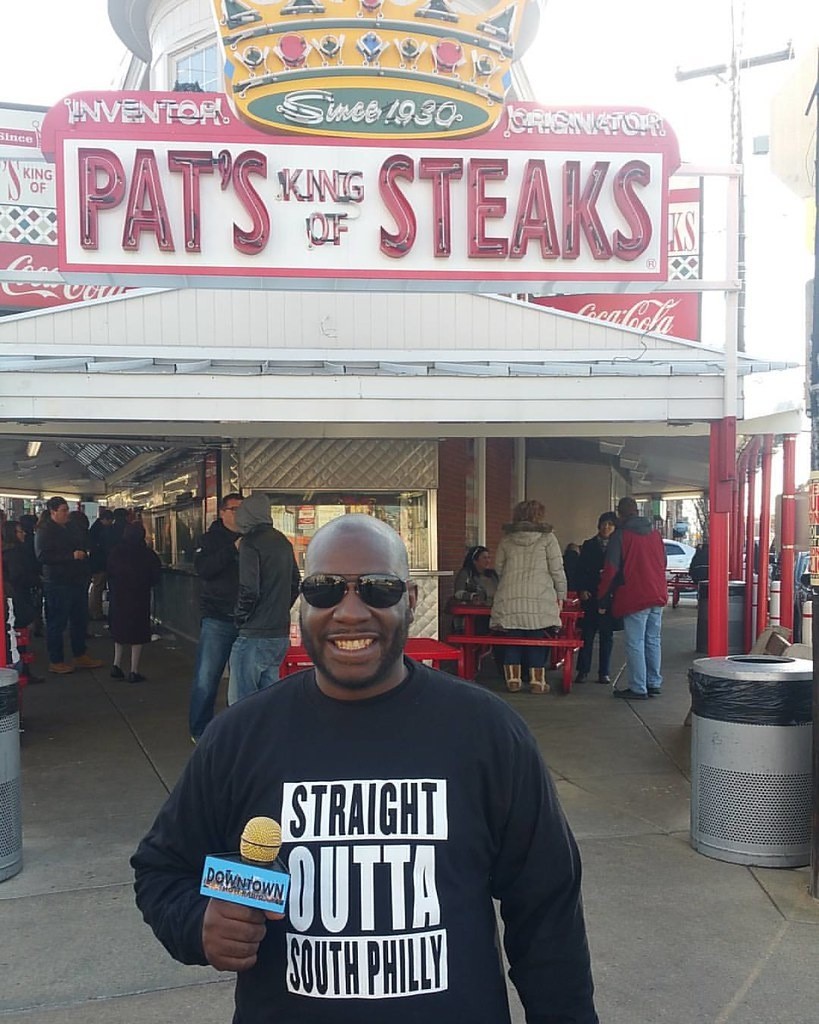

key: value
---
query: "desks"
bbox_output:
[671,570,689,582]
[453,606,584,669]
[279,637,466,680]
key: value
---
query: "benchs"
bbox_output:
[667,583,699,608]
[447,635,584,694]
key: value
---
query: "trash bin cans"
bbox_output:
[1,669,23,882]
[689,655,817,869]
[699,578,746,655]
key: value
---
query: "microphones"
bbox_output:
[199,815,293,914]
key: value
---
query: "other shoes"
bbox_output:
[48,662,74,674]
[613,689,648,700]
[576,672,587,683]
[27,674,46,683]
[600,675,611,684]
[129,672,148,682]
[111,665,124,680]
[647,688,662,696]
[74,655,104,668]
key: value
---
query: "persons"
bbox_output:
[0,497,131,683]
[597,497,668,696]
[564,544,584,592]
[454,546,529,680]
[360,578,400,595]
[131,515,599,1024]
[107,526,162,680]
[189,494,244,743]
[490,501,567,694]
[305,577,344,595]
[228,495,301,709]
[576,511,618,683]
[689,544,701,585]
[777,549,809,644]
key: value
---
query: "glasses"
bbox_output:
[224,507,237,513]
[299,573,413,609]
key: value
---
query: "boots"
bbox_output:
[530,667,551,695]
[504,664,522,692]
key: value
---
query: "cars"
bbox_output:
[662,539,700,592]
